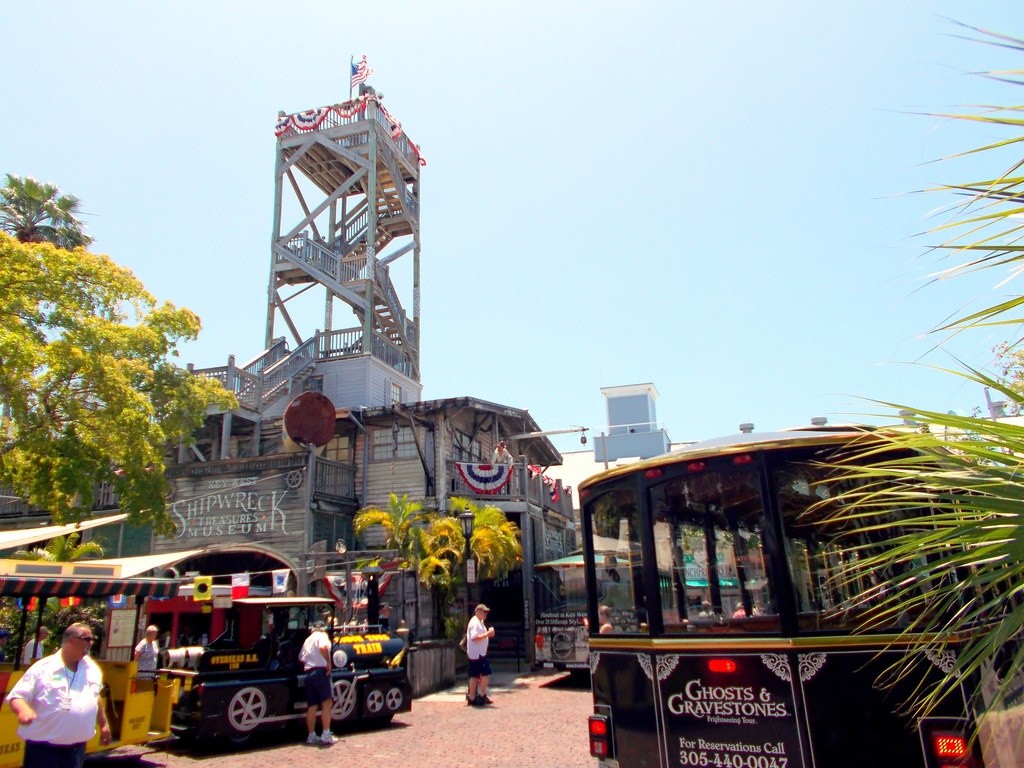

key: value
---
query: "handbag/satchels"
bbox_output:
[459,634,467,651]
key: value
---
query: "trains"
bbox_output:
[160,597,415,748]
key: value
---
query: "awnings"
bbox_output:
[685,579,739,590]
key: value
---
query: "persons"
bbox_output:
[298,621,338,744]
[9,623,111,768]
[24,626,49,665]
[0,630,9,662]
[734,603,745,618]
[134,625,159,679]
[466,604,494,706]
[181,625,197,646]
[699,600,713,619]
[491,441,513,465]
[582,606,612,633]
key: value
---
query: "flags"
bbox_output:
[350,60,373,87]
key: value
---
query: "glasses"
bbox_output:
[73,636,97,642]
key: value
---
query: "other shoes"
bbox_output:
[476,694,493,704]
[467,695,486,707]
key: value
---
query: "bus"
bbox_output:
[533,558,676,675]
[578,424,1020,768]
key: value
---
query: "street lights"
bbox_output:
[458,505,478,624]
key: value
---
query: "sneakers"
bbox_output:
[307,735,321,743]
[321,733,339,744]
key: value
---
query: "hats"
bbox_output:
[40,626,53,634]
[0,629,10,639]
[475,604,490,611]
